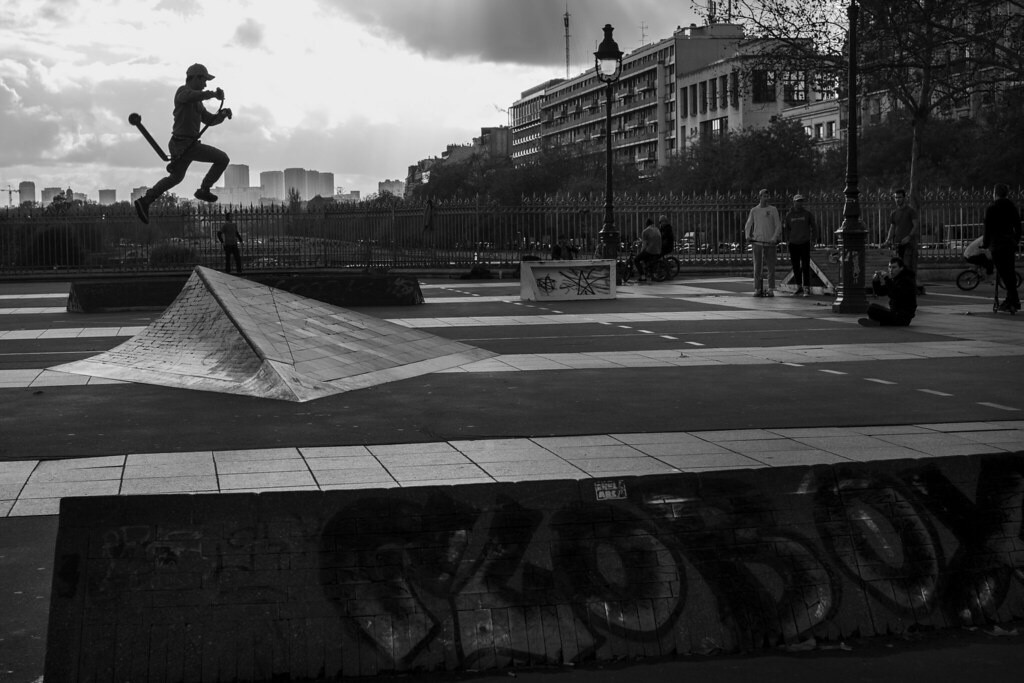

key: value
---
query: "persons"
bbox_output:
[884,188,921,273]
[784,194,819,297]
[134,63,232,225]
[744,188,782,298]
[982,184,1022,311]
[963,234,996,281]
[659,215,673,253]
[858,257,917,327]
[216,212,248,278]
[633,217,661,282]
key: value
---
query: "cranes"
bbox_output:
[0,185,20,208]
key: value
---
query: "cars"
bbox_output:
[675,231,942,253]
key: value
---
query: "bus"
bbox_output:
[942,223,984,253]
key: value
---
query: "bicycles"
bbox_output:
[956,249,1024,292]
[621,240,681,282]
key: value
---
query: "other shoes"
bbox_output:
[134,196,150,224]
[858,318,879,327]
[999,299,1021,310]
[754,289,762,297]
[794,288,804,295]
[637,277,647,282]
[194,189,218,202]
[768,289,774,297]
[984,274,996,283]
[803,288,810,298]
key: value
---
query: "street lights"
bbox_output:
[593,24,626,259]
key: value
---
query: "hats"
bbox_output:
[187,62,215,81]
[793,194,803,201]
